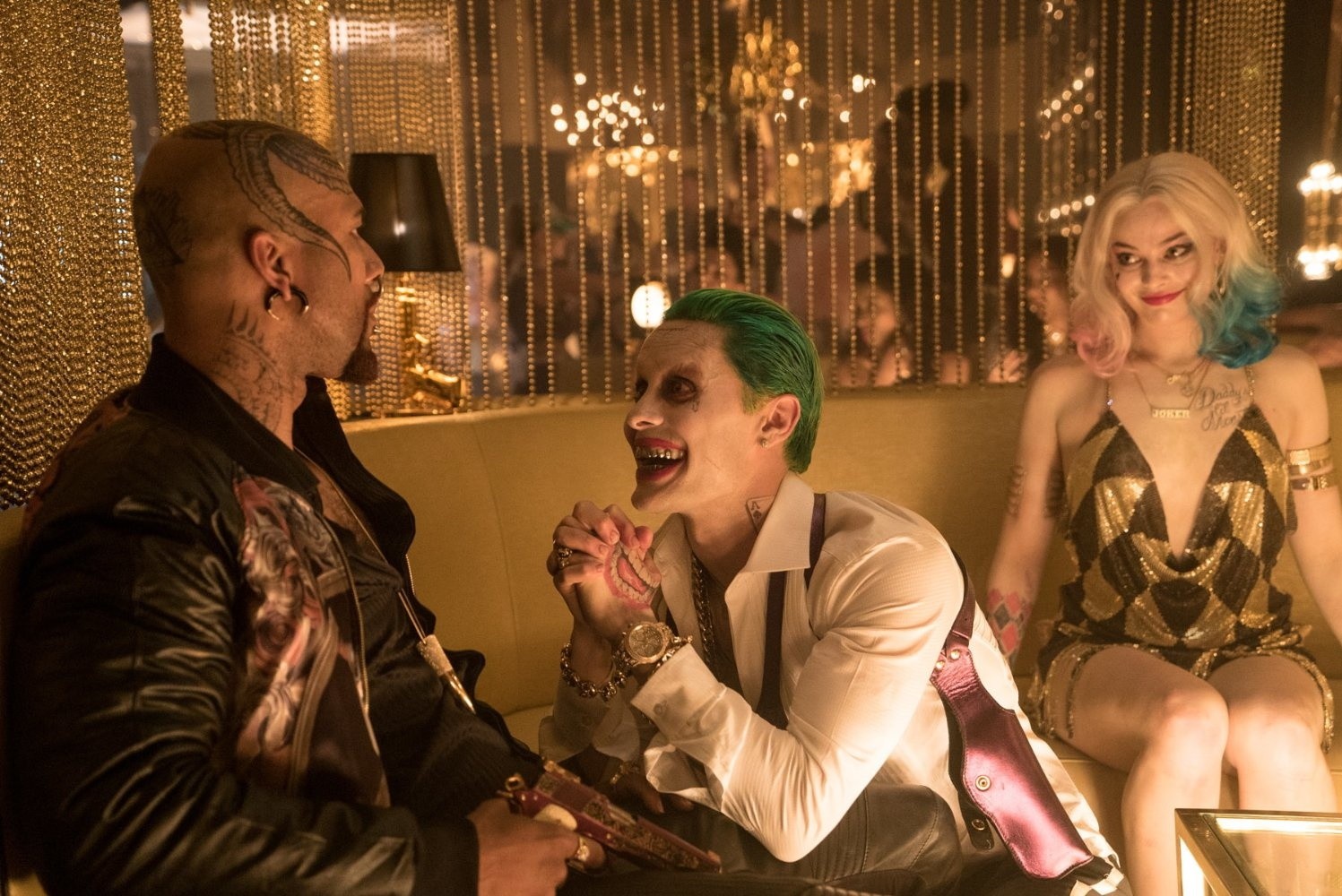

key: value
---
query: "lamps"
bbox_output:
[346,0,461,275]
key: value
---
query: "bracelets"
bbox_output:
[646,636,692,679]
[559,638,631,700]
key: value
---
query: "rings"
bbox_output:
[568,835,588,865]
[554,545,573,568]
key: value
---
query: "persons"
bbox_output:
[981,152,1341,896]
[1,121,964,896]
[489,222,1070,389]
[538,287,1133,896]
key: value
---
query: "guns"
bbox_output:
[494,758,720,879]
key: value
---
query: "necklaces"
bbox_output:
[293,446,475,715]
[1131,346,1208,386]
[1127,357,1212,418]
[691,553,722,684]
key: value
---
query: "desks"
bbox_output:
[1172,808,1342,896]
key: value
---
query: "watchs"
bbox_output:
[610,621,676,673]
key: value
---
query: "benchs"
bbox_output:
[0,373,1342,896]
